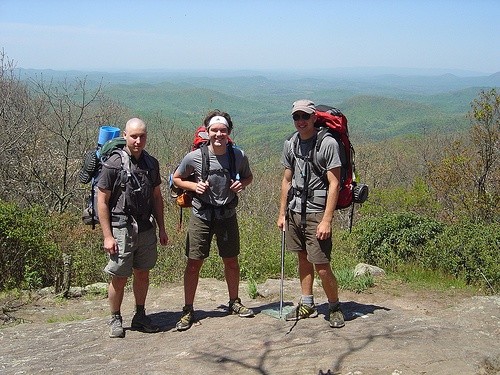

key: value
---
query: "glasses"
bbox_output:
[292,114,312,121]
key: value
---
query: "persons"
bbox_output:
[97,117,168,338]
[172,111,253,330]
[277,100,345,328]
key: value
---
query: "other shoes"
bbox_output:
[79,151,98,184]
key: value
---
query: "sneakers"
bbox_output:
[108,314,125,338]
[285,302,318,321]
[327,302,345,328]
[131,309,161,333]
[175,306,195,330]
[228,298,254,318]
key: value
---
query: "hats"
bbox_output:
[292,99,317,115]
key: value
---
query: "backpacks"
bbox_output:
[81,137,129,226]
[175,126,236,207]
[313,104,357,210]
